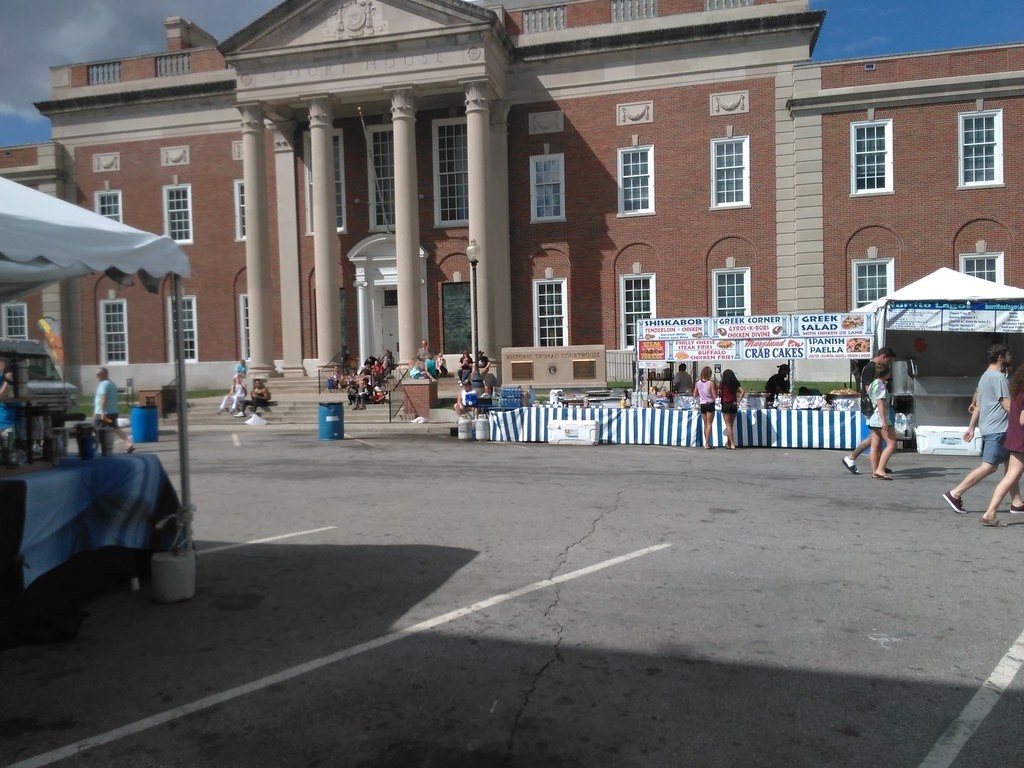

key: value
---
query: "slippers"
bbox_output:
[873,472,893,480]
[704,445,715,449]
[980,515,1007,527]
[726,441,736,450]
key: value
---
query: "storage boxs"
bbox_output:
[522,391,530,407]
[916,425,982,456]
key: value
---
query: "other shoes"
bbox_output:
[351,405,366,410]
[458,380,463,387]
[884,467,893,473]
[842,456,860,475]
[234,411,245,417]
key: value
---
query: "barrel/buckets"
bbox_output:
[130,405,158,443]
[319,402,344,440]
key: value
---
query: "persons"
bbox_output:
[216,374,247,415]
[330,349,394,410]
[765,363,790,402]
[624,390,628,400]
[471,350,491,381]
[233,379,270,417]
[717,369,745,449]
[234,359,247,372]
[0,360,16,450]
[457,350,474,385]
[693,366,717,449]
[409,340,438,383]
[453,378,492,416]
[842,345,897,480]
[92,367,136,453]
[435,353,448,377]
[674,363,693,393]
[942,342,1024,528]
[799,387,810,395]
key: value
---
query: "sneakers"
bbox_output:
[942,490,968,513]
[1010,502,1024,514]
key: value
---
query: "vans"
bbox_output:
[0,337,79,414]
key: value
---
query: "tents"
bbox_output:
[848,265,1024,387]
[0,169,192,537]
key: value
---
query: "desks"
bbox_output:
[488,405,863,450]
[463,404,521,410]
[0,450,191,656]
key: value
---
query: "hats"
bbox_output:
[233,372,244,381]
[777,364,790,371]
[462,379,471,385]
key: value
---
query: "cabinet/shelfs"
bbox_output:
[647,361,674,404]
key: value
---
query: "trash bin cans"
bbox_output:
[858,402,896,453]
[318,400,345,441]
[129,406,160,443]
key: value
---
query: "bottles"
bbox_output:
[501,387,522,407]
[584,398,588,407]
[621,399,625,409]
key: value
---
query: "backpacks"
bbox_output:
[861,381,883,416]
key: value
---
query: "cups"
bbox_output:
[75,422,99,460]
[52,427,69,455]
[99,427,114,457]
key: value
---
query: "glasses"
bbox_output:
[96,373,106,377]
